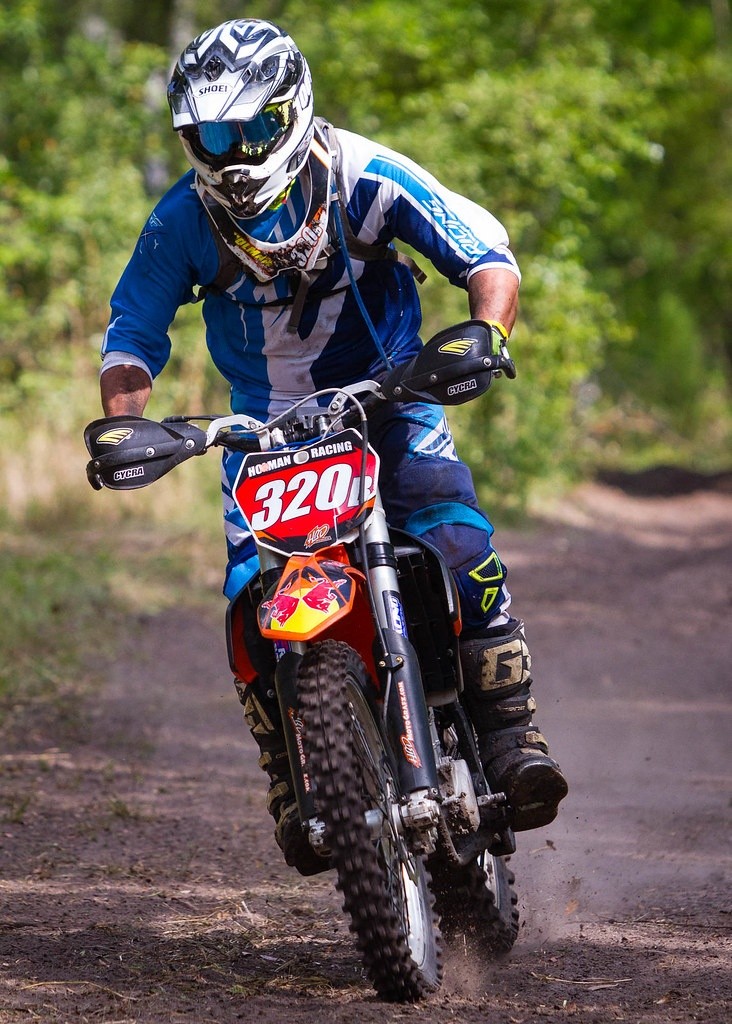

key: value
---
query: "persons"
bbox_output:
[99,15,569,876]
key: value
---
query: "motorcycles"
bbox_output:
[85,322,561,991]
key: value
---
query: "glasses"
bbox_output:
[196,101,292,157]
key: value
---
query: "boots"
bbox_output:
[234,672,339,877]
[453,617,569,831]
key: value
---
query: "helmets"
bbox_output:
[166,19,315,220]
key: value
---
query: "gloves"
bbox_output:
[481,319,515,381]
